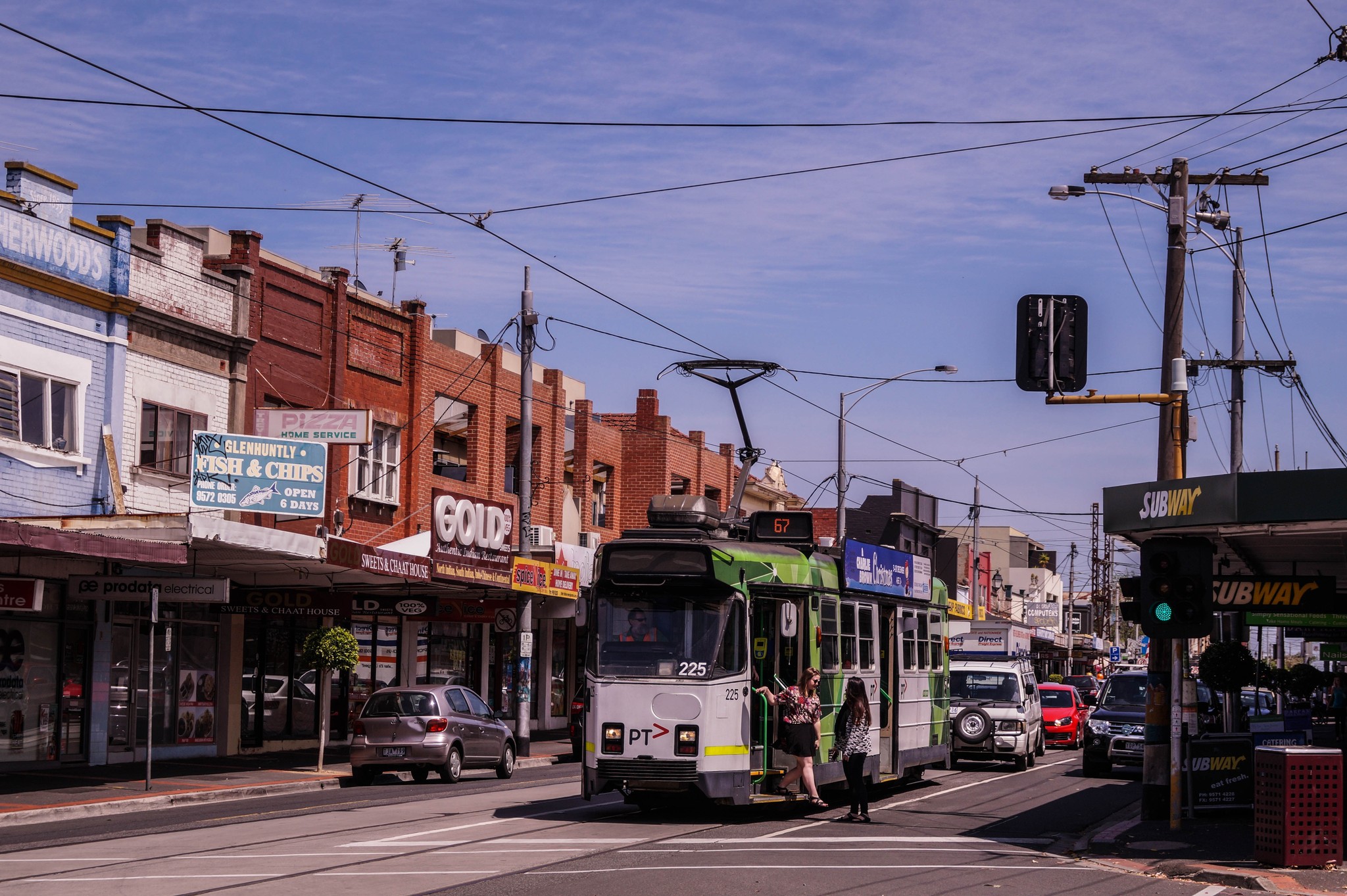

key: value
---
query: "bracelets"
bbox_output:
[766,687,768,691]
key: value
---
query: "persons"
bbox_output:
[756,668,828,807]
[1326,676,1346,741]
[611,607,668,642]
[751,658,759,746]
[832,676,872,823]
[841,652,851,669]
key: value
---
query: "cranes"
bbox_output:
[1090,502,1118,682]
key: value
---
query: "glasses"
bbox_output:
[809,678,820,684]
[634,617,647,622]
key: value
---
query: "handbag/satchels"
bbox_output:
[771,736,789,750]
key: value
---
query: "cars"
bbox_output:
[58,649,375,748]
[1187,676,1285,732]
[353,682,523,784]
[1034,680,1087,749]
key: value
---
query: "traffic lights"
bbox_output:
[1015,288,1088,392]
[1118,533,1213,638]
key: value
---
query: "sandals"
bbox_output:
[774,785,797,796]
[807,796,829,808]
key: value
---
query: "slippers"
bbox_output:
[836,812,856,822]
[851,813,871,823]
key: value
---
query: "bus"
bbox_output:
[572,495,951,812]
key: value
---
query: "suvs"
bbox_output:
[1083,667,1153,777]
[1062,672,1098,704]
[948,651,1048,766]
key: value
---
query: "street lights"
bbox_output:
[834,362,957,546]
[973,472,1003,623]
[1055,184,1247,481]
[1068,541,1082,676]
